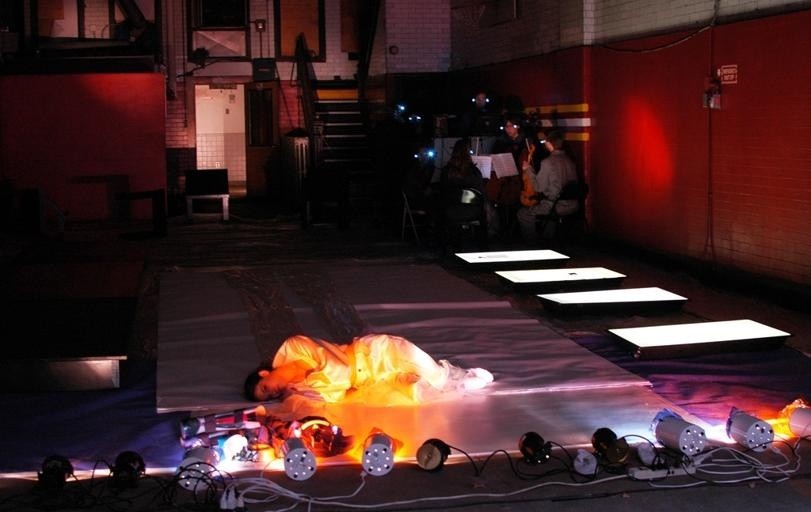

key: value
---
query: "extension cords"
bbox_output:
[177,498,249,512]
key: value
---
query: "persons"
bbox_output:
[433,91,580,236]
[244,335,495,403]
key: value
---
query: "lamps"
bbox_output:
[37,400,811,491]
